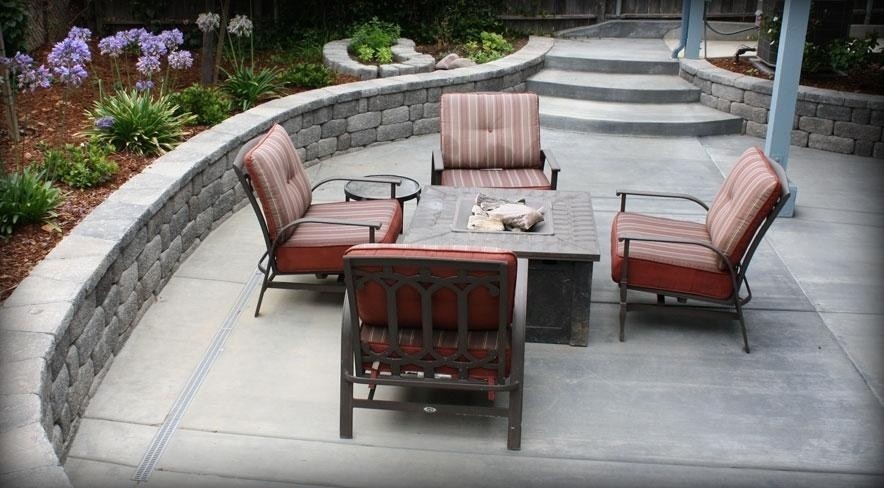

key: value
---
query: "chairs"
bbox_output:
[611,155,790,353]
[340,255,530,450]
[232,133,403,317]
[431,92,561,190]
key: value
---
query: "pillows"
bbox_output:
[245,123,312,247]
[440,91,541,169]
[343,243,517,331]
[706,146,782,272]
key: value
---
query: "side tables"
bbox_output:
[345,173,420,233]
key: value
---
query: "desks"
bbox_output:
[403,185,600,346]
